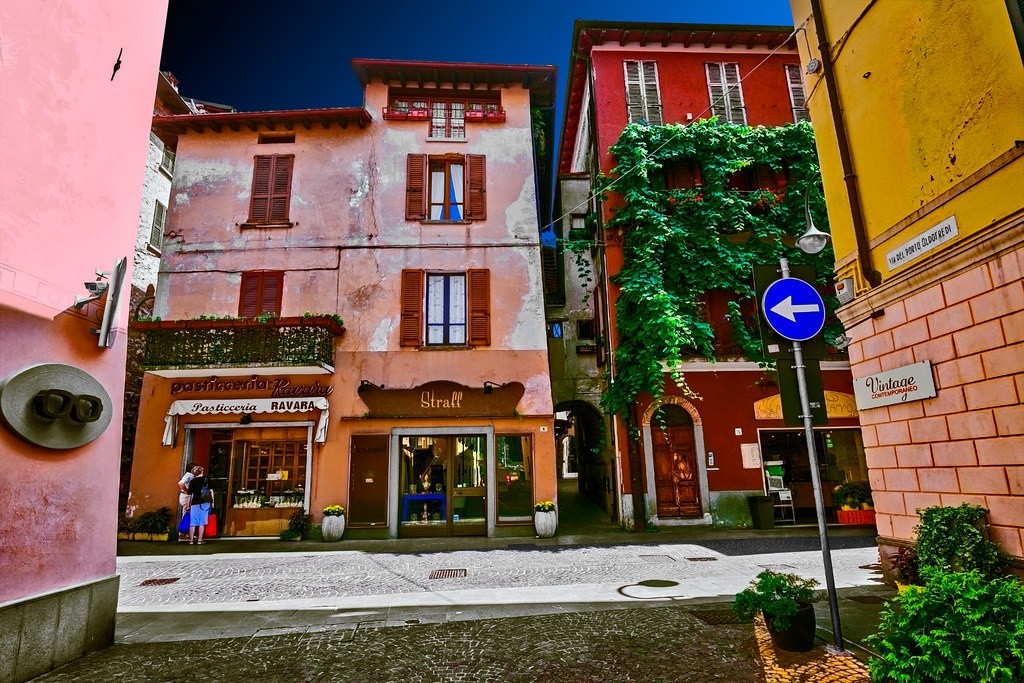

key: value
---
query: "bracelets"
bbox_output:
[212,500,214,502]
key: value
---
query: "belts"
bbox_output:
[180,492,192,495]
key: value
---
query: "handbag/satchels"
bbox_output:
[201,477,212,501]
[179,508,191,535]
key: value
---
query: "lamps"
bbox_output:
[358,379,384,392]
[240,412,255,425]
[483,381,505,394]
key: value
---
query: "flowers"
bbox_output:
[322,505,347,518]
[534,500,557,513]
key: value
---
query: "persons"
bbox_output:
[178,463,198,538]
[187,465,214,544]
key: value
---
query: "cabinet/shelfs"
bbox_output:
[768,488,796,525]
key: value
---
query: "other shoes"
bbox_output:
[193,535,198,539]
[180,536,189,539]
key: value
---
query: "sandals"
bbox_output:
[189,541,194,545]
[197,541,206,545]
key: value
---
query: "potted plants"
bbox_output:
[830,480,876,510]
[281,508,312,542]
[118,507,175,542]
[732,569,830,652]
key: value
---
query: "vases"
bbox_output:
[321,515,345,541]
[534,511,557,538]
